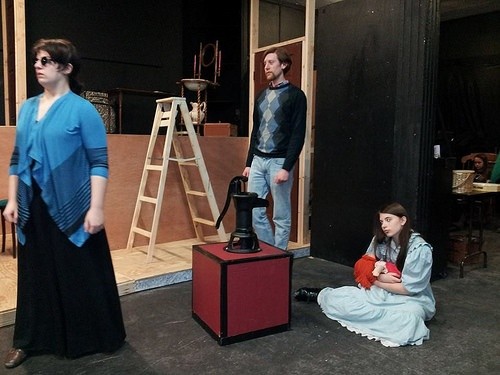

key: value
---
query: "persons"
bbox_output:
[3,38,127,367]
[243,47,307,251]
[464,154,495,182]
[294,203,436,347]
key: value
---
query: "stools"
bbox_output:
[0,198,16,259]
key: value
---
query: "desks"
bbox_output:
[448,189,500,279]
[191,239,294,347]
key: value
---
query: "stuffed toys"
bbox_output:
[354,254,400,288]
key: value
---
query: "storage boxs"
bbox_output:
[204,122,238,138]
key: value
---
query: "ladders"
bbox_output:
[127,97,228,264]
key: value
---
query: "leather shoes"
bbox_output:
[4,348,27,367]
[293,288,322,301]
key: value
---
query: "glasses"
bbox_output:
[34,57,54,65]
